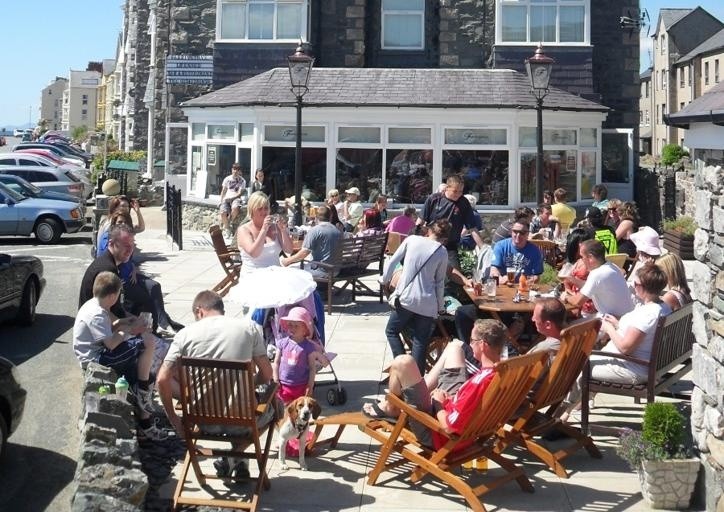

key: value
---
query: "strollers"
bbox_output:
[224,266,352,407]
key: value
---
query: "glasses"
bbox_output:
[512,229,530,235]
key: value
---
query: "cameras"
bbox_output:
[268,216,278,224]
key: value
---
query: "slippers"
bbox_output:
[361,401,397,424]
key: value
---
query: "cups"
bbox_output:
[486,278,496,302]
[139,311,152,330]
[506,267,515,284]
[543,228,551,238]
[473,282,482,296]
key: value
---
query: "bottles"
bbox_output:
[518,269,528,300]
[553,287,559,297]
[113,377,129,402]
[98,384,110,398]
[529,286,540,299]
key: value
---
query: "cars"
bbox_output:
[0,357,27,448]
[0,126,95,243]
[0,251,46,326]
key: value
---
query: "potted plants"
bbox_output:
[639,402,698,511]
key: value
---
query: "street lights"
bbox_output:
[522,39,562,215]
[286,39,317,231]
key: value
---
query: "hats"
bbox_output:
[345,187,360,196]
[630,226,661,256]
[280,306,314,339]
[584,207,602,218]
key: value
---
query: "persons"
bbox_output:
[557,224,693,422]
[523,299,566,394]
[234,192,292,285]
[360,319,509,456]
[74,271,169,443]
[281,206,342,280]
[78,197,186,378]
[285,195,310,230]
[156,291,284,482]
[486,182,641,288]
[252,167,279,215]
[378,175,487,382]
[219,163,246,239]
[327,190,388,265]
[271,307,317,450]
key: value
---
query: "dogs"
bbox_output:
[274,396,321,471]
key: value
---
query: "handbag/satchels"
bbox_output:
[387,293,401,310]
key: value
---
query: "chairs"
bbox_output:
[209,208,700,316]
[174,354,283,511]
[489,318,610,478]
[578,302,695,447]
[306,348,560,511]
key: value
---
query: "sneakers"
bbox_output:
[137,425,168,442]
[213,457,235,486]
[236,462,250,481]
[129,382,155,414]
[224,227,232,239]
[156,324,176,337]
